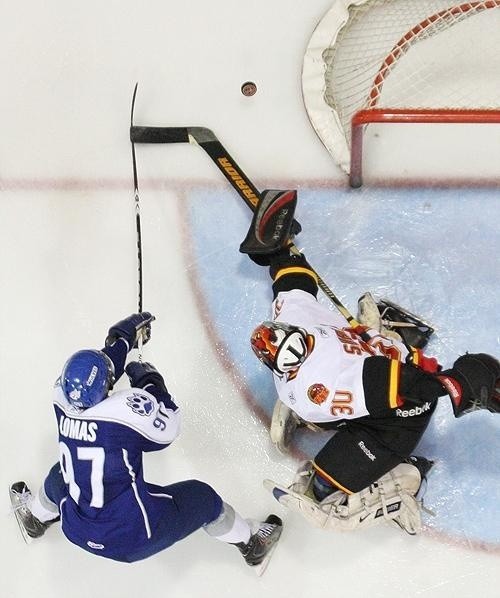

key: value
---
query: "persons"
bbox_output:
[238,189,500,536]
[10,312,283,567]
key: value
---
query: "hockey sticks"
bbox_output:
[132,85,145,362]
[129,125,364,336]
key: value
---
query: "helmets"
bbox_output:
[249,320,307,374]
[59,348,115,409]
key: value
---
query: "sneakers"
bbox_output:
[376,306,430,350]
[409,453,430,498]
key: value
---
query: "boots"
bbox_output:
[227,514,282,567]
[10,481,59,540]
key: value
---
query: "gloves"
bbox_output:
[238,187,303,265]
[124,359,169,405]
[432,351,500,419]
[105,311,153,351]
